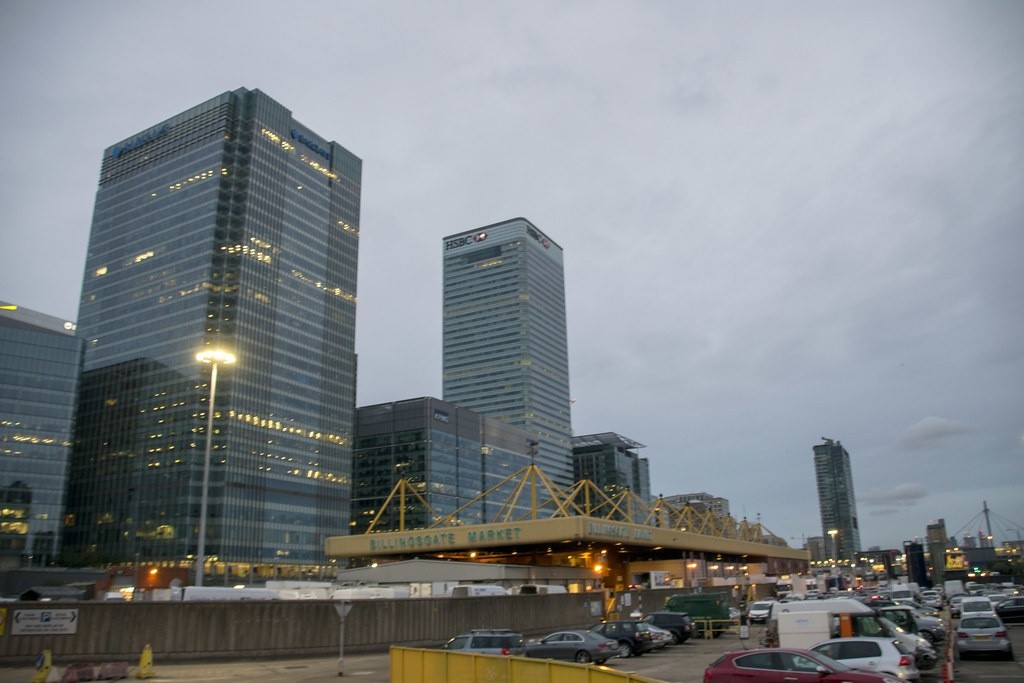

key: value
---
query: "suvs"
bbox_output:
[749,601,777,625]
[793,638,921,681]
[441,628,528,656]
[703,648,908,683]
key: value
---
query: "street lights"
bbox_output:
[191,345,237,587]
[827,529,840,589]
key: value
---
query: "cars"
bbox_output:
[590,609,697,657]
[944,580,1024,659]
[728,607,740,620]
[770,580,944,670]
[525,631,623,666]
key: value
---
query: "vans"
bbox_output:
[664,593,732,638]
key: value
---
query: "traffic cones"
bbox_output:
[28,650,53,683]
[136,643,157,680]
